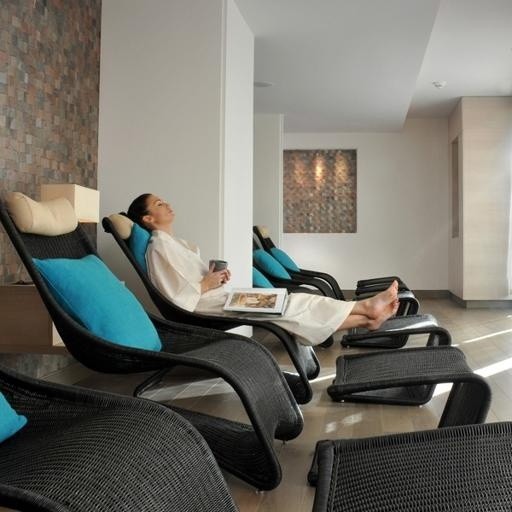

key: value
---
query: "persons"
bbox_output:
[127,193,400,346]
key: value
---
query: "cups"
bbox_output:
[209,260,227,283]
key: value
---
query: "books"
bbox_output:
[223,288,288,318]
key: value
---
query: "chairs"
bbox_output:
[0,202,305,491]
[253,232,337,305]
[0,362,237,511]
[101,212,320,405]
[252,263,335,349]
[253,225,347,305]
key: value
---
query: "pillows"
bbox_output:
[33,253,164,354]
[252,268,274,289]
[257,221,268,240]
[254,247,292,280]
[269,242,304,273]
[8,189,79,237]
[128,225,151,274]
[108,212,135,242]
